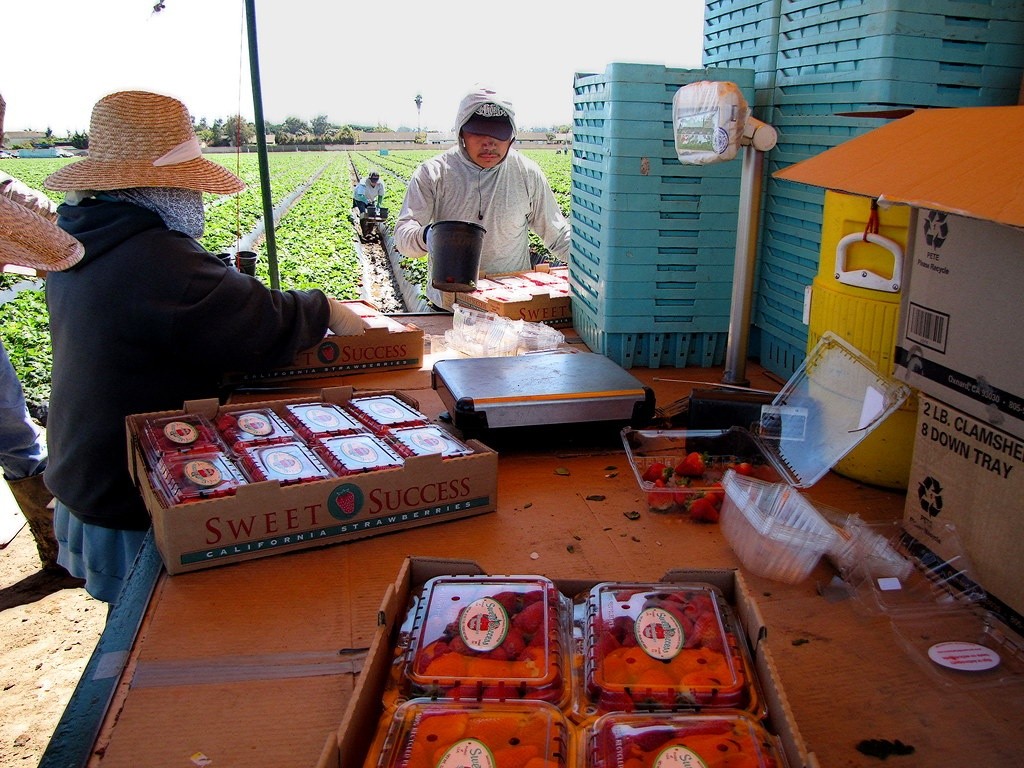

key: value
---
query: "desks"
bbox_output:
[36,312,1024,768]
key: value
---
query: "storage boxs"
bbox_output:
[123,0,1024,768]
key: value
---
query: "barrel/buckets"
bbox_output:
[215,251,258,278]
[428,219,487,291]
[805,190,921,489]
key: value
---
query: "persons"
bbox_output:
[42,92,369,620]
[393,87,571,313]
[353,171,385,240]
[0,100,62,564]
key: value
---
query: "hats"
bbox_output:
[43,91,245,195]
[369,172,380,182]
[0,192,84,273]
[462,90,513,141]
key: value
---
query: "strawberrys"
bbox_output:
[641,451,752,522]
[137,394,478,507]
[366,581,781,768]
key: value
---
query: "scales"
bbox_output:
[430,80,780,451]
